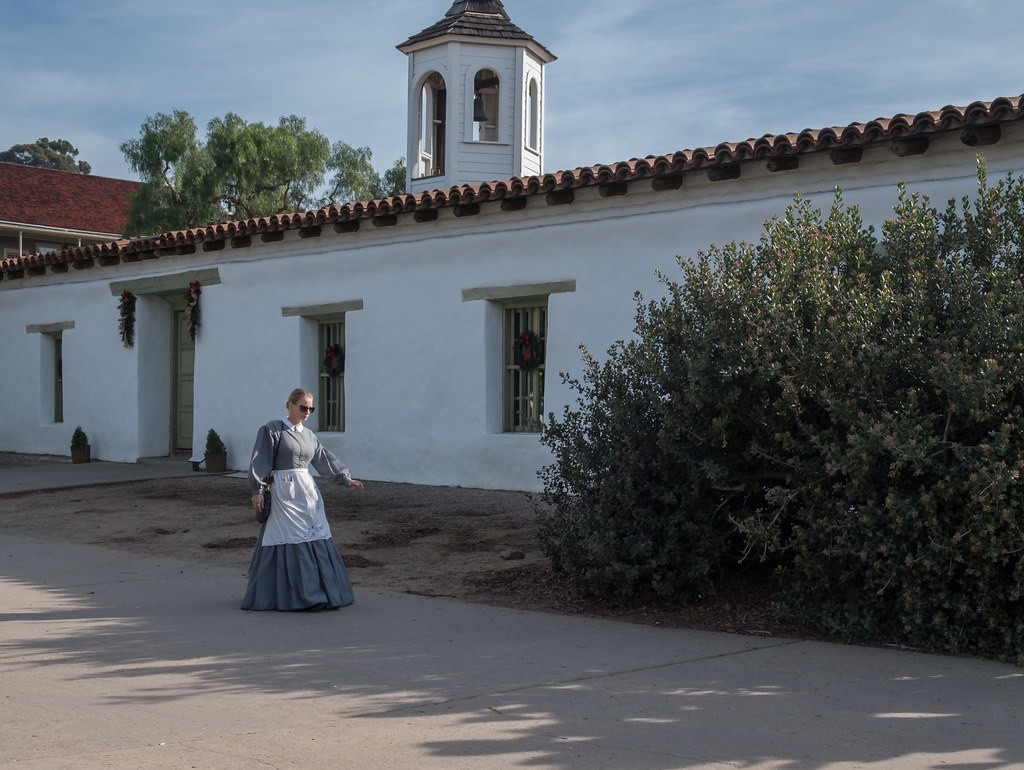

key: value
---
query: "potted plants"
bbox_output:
[204,428,227,472]
[70,426,90,462]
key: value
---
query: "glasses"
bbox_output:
[293,402,315,413]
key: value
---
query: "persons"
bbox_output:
[239,388,363,611]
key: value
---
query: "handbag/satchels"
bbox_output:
[256,489,272,523]
